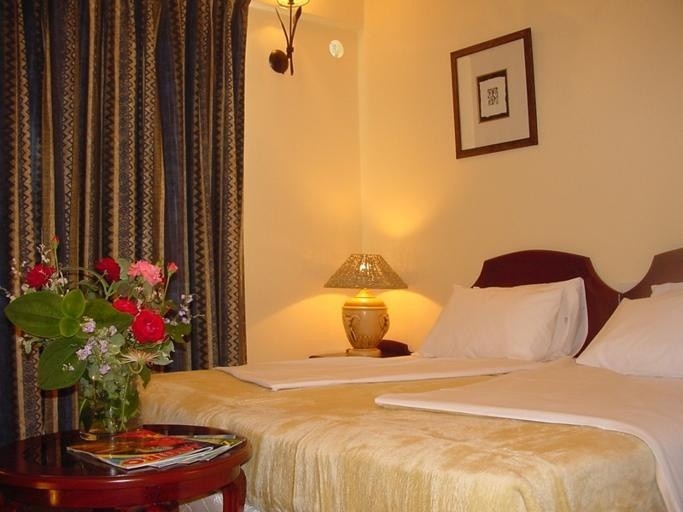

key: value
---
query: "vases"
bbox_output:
[71,376,139,439]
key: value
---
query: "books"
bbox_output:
[66,426,246,471]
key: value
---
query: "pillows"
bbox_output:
[415,276,682,380]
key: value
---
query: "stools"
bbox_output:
[0,240,202,424]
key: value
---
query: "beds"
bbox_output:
[134,248,682,512]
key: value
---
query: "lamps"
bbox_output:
[324,252,408,357]
[267,1,308,78]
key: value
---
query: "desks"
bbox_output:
[3,426,251,511]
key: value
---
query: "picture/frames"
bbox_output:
[448,28,540,162]
